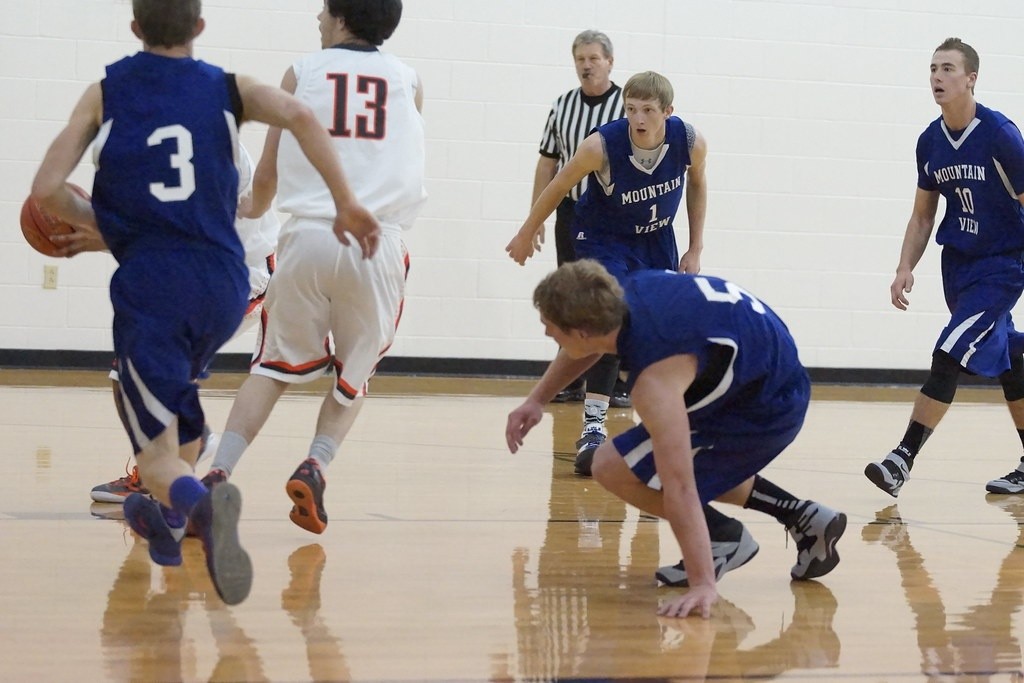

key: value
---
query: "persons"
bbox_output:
[864,37,1024,498]
[30,0,381,605]
[505,258,848,620]
[530,29,633,409]
[184,0,429,537]
[505,72,707,475]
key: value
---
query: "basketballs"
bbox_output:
[20,182,91,257]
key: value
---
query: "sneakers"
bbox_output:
[653,519,761,587]
[609,389,632,407]
[91,461,146,503]
[782,499,847,580]
[287,459,328,533]
[866,446,914,497]
[201,469,225,490]
[122,494,188,567]
[553,390,586,402]
[194,483,253,604]
[574,431,607,475]
[986,457,1024,492]
